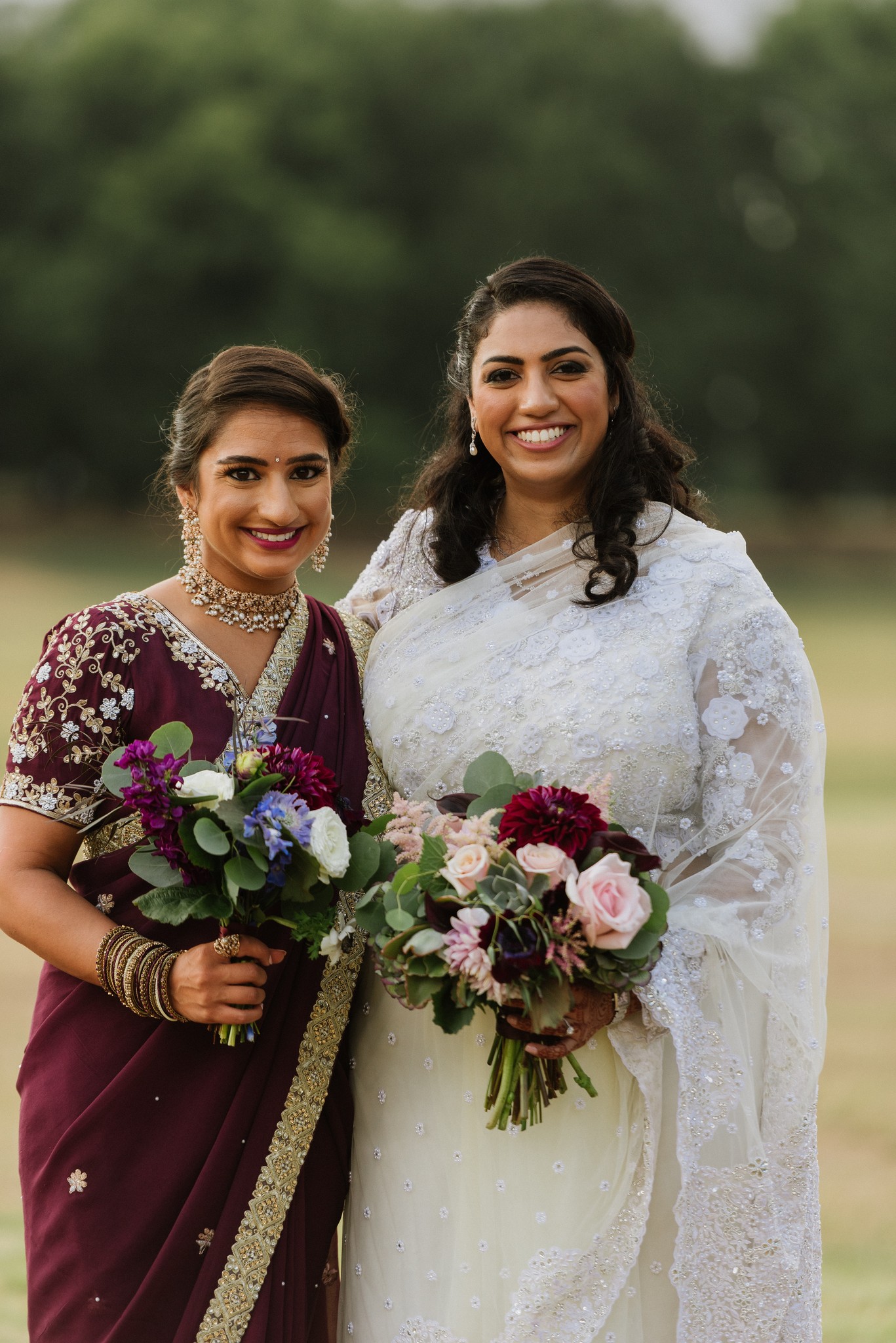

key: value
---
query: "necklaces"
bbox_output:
[178,558,299,634]
[493,493,544,577]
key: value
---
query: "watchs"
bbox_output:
[606,990,631,1029]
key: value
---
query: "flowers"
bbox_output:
[98,718,670,1133]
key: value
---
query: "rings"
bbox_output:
[213,933,241,959]
[561,1017,576,1036]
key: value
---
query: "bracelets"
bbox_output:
[95,925,190,1024]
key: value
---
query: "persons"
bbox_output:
[328,256,832,1343]
[0,344,395,1342]
